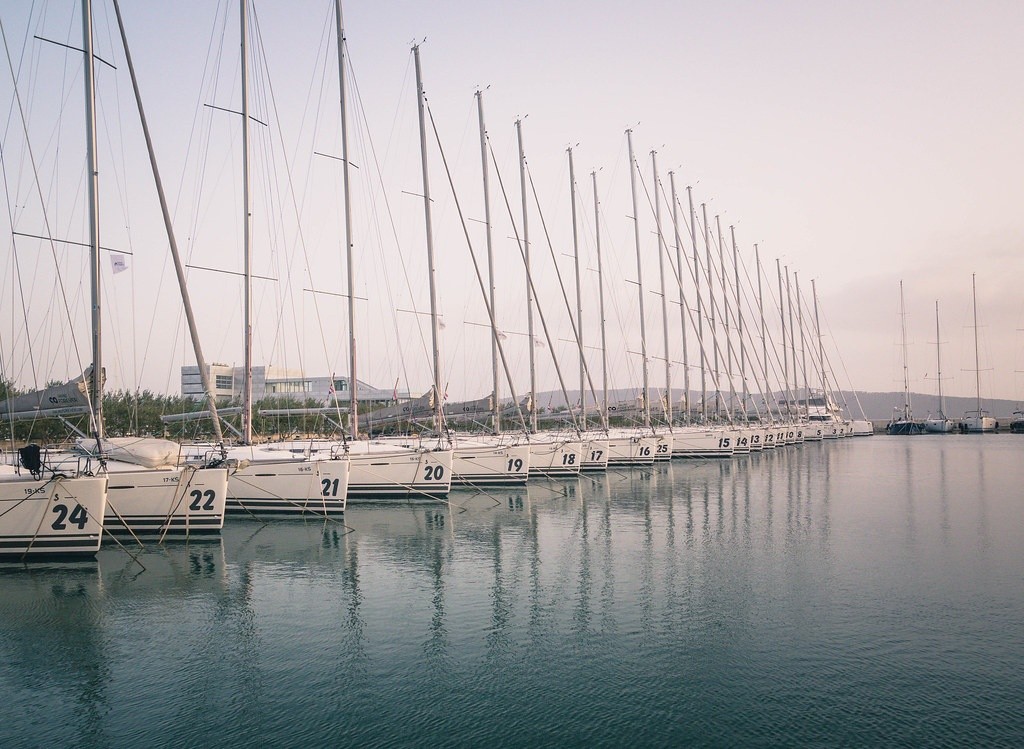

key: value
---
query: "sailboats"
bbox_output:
[0,0,1024,559]
